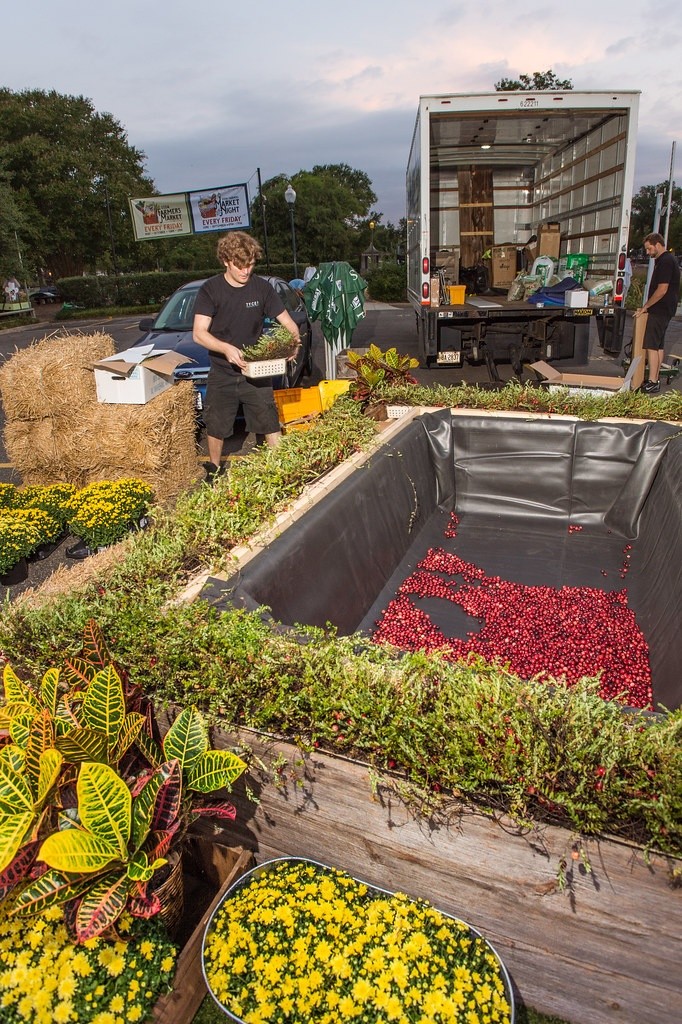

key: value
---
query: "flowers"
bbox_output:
[0,624,514,1024]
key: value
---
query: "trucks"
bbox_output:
[404,89,642,371]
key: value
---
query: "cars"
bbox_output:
[29,292,60,304]
[129,277,313,428]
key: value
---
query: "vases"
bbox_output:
[141,827,254,1024]
[0,555,28,586]
[66,514,148,558]
[145,848,188,944]
[0,478,156,586]
[26,525,70,564]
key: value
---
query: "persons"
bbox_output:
[632,233,680,392]
[192,232,302,486]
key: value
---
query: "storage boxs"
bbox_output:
[631,308,648,386]
[565,291,588,308]
[492,223,560,288]
[446,285,467,304]
[89,344,195,406]
[336,348,382,380]
[529,356,642,399]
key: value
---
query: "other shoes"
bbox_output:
[203,461,224,482]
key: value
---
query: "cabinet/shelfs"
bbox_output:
[201,857,514,1024]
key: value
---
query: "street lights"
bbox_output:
[285,185,298,281]
[369,222,376,269]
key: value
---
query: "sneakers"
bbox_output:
[636,378,660,392]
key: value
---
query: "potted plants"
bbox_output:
[242,325,298,378]
